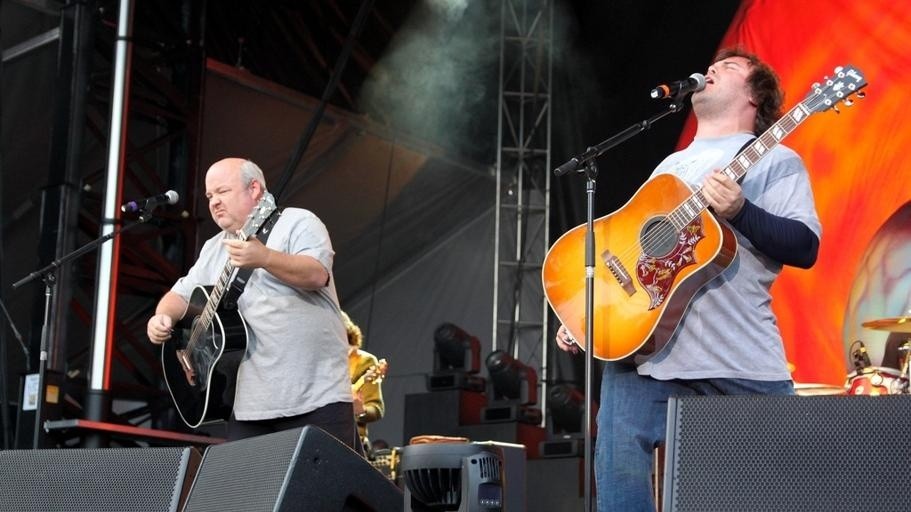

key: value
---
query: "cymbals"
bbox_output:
[863,318,911,335]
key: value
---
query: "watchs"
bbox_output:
[356,410,369,424]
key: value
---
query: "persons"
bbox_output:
[555,48,823,511]
[145,156,356,456]
[340,308,386,458]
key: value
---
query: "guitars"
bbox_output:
[541,65,868,369]
[161,191,278,427]
[354,358,388,393]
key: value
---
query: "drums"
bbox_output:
[845,367,909,395]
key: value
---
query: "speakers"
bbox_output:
[180,423,403,512]
[0,445,202,511]
[661,393,911,512]
[401,441,526,512]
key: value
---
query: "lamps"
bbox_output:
[424,322,597,459]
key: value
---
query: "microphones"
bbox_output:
[860,342,871,367]
[649,71,706,99]
[120,189,180,212]
